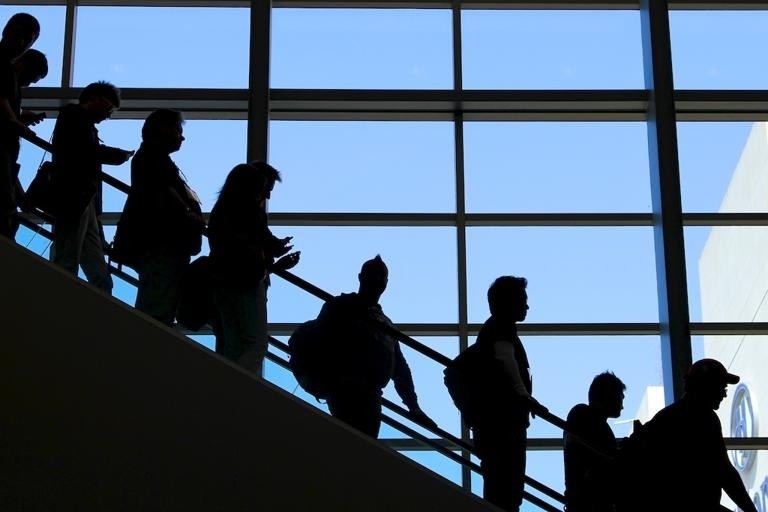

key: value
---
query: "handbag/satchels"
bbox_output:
[25,159,54,215]
[177,254,217,332]
[110,193,138,272]
[287,317,332,400]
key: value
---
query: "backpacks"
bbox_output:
[610,420,652,512]
[444,343,482,429]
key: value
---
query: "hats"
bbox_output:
[688,357,740,385]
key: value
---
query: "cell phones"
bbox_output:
[28,112,46,124]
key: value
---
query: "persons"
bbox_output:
[638,361,758,510]
[318,253,438,447]
[475,276,551,512]
[129,109,203,331]
[7,48,47,200]
[208,160,271,377]
[249,160,302,290]
[564,371,630,511]
[0,12,38,239]
[49,81,134,299]
